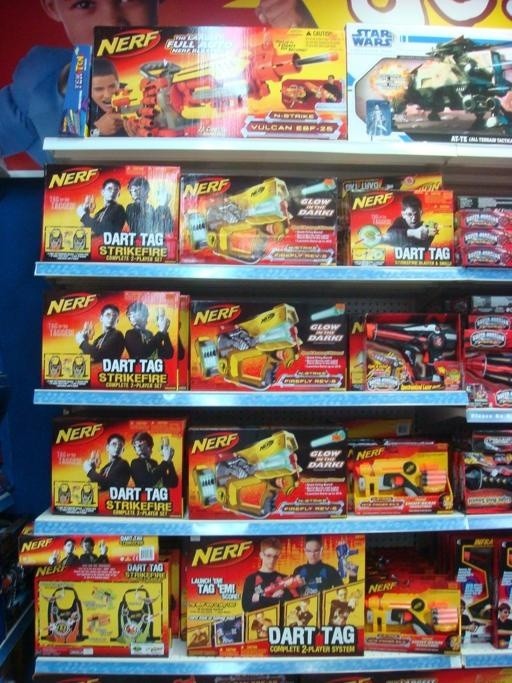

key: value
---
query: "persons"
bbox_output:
[79,175,170,234]
[3,0,158,171]
[241,536,358,614]
[91,58,128,136]
[460,600,512,645]
[322,76,341,103]
[50,536,109,566]
[384,196,439,248]
[78,300,173,385]
[84,432,179,516]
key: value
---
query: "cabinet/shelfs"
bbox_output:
[34,137,512,683]
[1,372,32,670]
